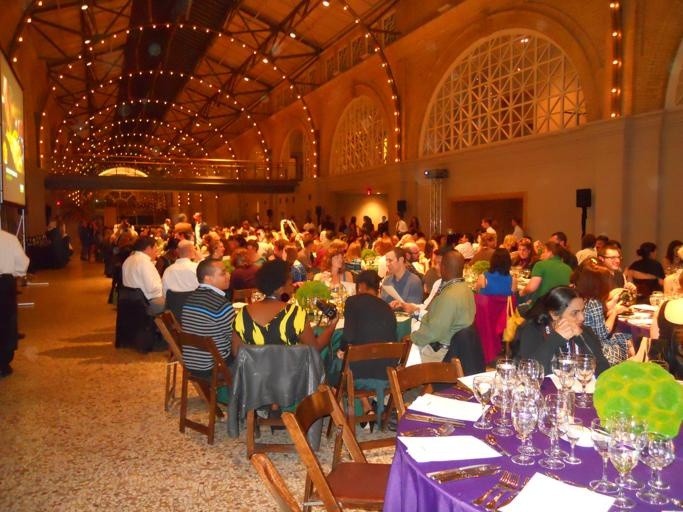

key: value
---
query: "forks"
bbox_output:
[497,476,533,509]
[486,473,521,510]
[474,470,511,507]
[402,424,455,437]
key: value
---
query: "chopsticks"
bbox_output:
[405,413,465,426]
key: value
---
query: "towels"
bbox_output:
[399,434,504,463]
[408,393,489,422]
[458,369,499,395]
[496,471,615,511]
[546,370,596,394]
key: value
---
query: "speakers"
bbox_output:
[267,209,272,217]
[315,206,322,216]
[576,189,591,207]
[397,200,406,211]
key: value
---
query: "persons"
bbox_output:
[161,239,200,305]
[518,241,575,312]
[472,249,518,296]
[282,245,306,282]
[550,231,578,269]
[517,286,609,378]
[569,265,635,367]
[605,240,665,286]
[598,244,637,306]
[381,248,423,309]
[423,268,439,294]
[180,258,236,377]
[122,236,165,313]
[575,234,599,266]
[371,249,476,408]
[231,259,340,436]
[629,242,665,294]
[0,228,30,379]
[661,240,683,274]
[77,211,536,255]
[595,235,608,253]
[636,304,683,380]
[389,244,454,331]
[329,269,399,433]
[231,247,260,289]
[313,249,353,284]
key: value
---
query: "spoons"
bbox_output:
[485,434,511,458]
[433,392,475,400]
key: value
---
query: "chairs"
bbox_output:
[113,286,168,353]
[108,259,123,304]
[178,332,232,445]
[234,289,261,302]
[387,358,466,421]
[327,339,413,439]
[100,240,122,277]
[252,383,392,512]
[246,346,322,460]
[251,452,303,512]
[473,294,517,356]
[423,322,483,394]
[155,310,224,419]
[623,268,659,290]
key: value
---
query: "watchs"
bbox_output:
[656,275,659,280]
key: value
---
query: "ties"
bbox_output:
[397,221,399,230]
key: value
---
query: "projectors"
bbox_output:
[424,168,448,178]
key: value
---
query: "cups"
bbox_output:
[551,352,594,408]
[251,290,264,305]
[539,394,582,469]
[591,417,619,493]
[495,359,543,465]
[653,291,663,306]
[474,377,496,430]
[522,269,531,277]
[611,410,647,509]
[636,431,676,505]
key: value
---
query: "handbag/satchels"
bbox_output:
[502,295,525,341]
[603,255,622,261]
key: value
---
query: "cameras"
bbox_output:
[314,299,338,320]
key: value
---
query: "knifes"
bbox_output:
[432,466,501,481]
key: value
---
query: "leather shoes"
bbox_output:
[18,332,26,338]
[1,366,13,377]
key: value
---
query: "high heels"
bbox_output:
[360,410,375,433]
